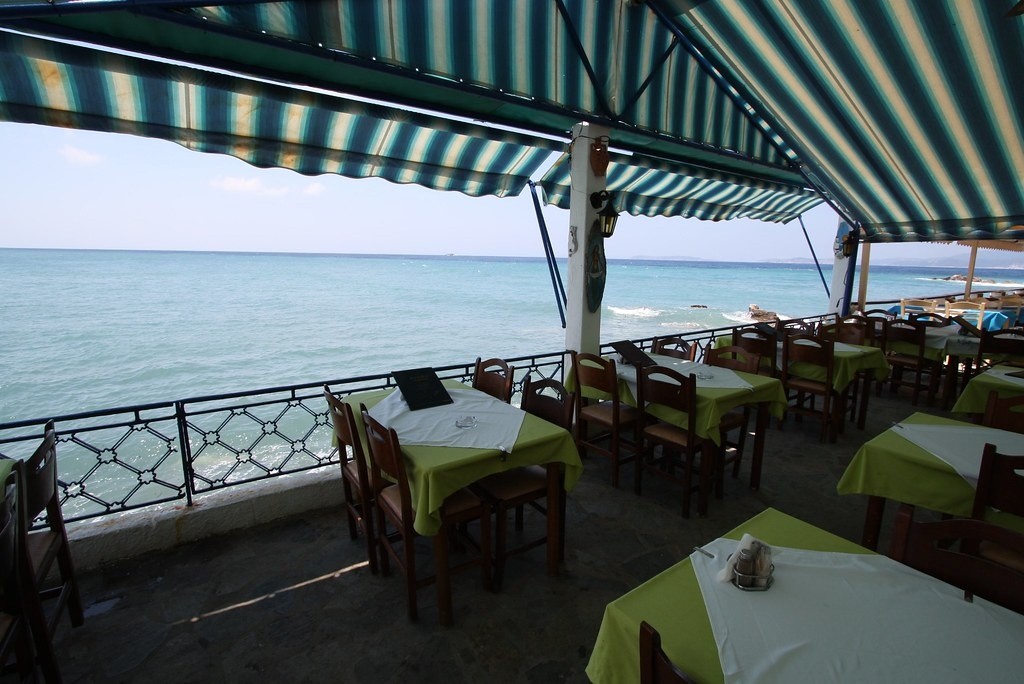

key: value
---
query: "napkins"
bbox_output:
[716,533,781,582]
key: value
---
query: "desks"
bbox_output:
[583,508,1024,683]
[337,307,1024,626]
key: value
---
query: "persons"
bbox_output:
[589,245,603,273]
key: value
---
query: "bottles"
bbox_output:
[737,541,772,588]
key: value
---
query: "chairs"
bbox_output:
[459,357,512,541]
[637,619,697,684]
[569,293,1024,613]
[0,418,83,684]
[323,385,425,575]
[360,402,495,624]
[465,373,573,596]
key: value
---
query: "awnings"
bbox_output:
[0,0,841,328]
[645,0,1024,242]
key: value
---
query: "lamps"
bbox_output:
[590,189,620,237]
[842,231,858,258]
